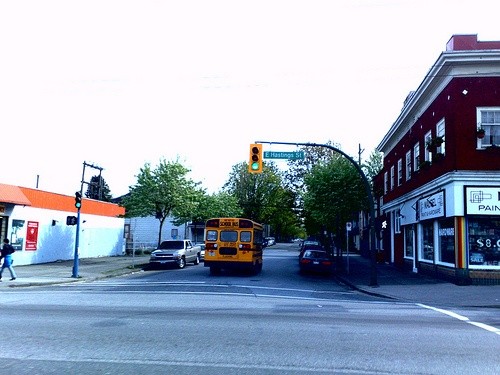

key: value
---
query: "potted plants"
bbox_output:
[419,137,444,169]
[478,129,485,138]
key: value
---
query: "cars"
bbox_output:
[298,250,334,276]
[195,243,206,262]
[265,237,275,247]
[297,240,325,261]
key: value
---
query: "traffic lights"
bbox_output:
[75,191,81,208]
[248,143,263,174]
[66,216,77,226]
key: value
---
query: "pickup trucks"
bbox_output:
[148,240,202,269]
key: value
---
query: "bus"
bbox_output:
[202,217,269,275]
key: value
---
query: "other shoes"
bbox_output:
[0,278,3,282]
[9,277,16,281]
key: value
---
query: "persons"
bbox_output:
[0,238,16,280]
